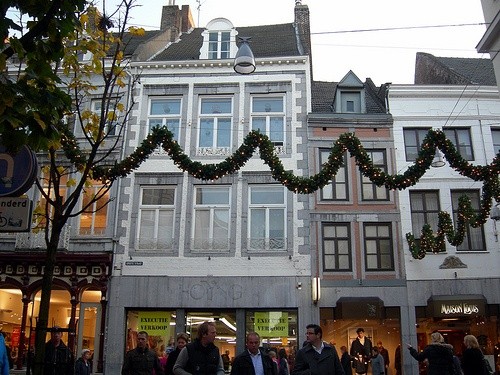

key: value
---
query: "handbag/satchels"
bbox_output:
[479,348,493,372]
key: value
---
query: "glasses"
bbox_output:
[305,332,315,336]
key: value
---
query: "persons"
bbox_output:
[121,322,230,375]
[0,333,10,375]
[371,341,389,375]
[230,332,291,375]
[293,324,346,375]
[395,343,402,375]
[406,333,462,375]
[340,346,366,375]
[461,335,490,375]
[350,328,372,357]
[44,326,75,375]
[75,349,92,375]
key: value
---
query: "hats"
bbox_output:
[82,349,90,354]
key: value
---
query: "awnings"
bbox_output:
[427,295,488,315]
[336,297,384,319]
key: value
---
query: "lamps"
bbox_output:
[432,146,447,167]
[234,36,257,75]
